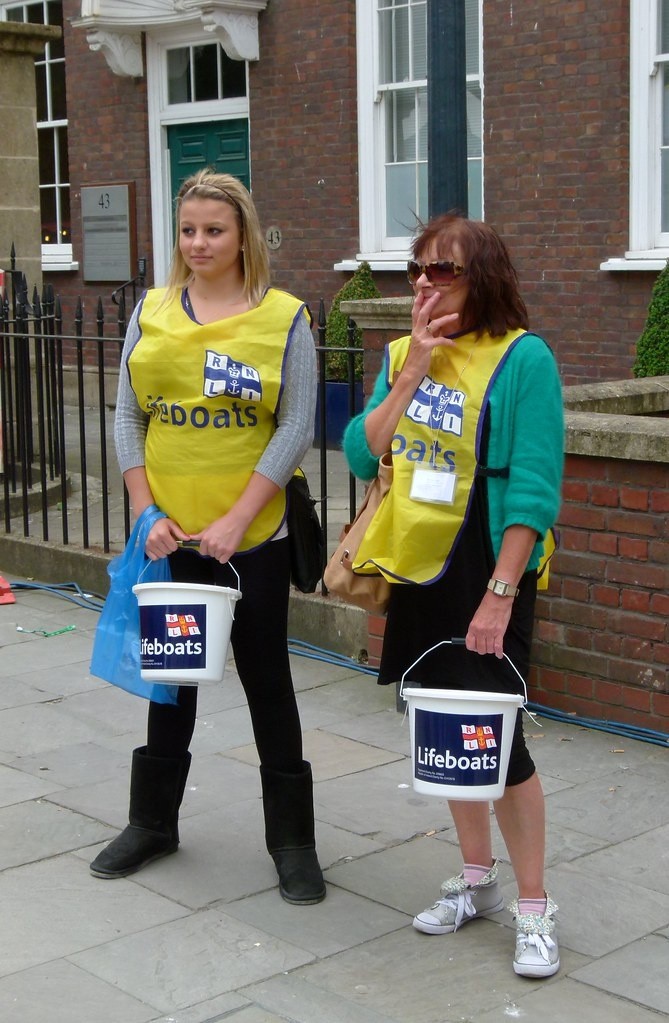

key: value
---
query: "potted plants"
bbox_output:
[312,260,381,447]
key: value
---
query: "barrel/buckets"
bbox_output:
[398,639,528,801]
[132,538,242,685]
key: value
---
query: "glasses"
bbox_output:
[405,259,470,287]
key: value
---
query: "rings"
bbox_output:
[214,552,220,558]
[425,326,432,333]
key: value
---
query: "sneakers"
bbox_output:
[510,891,559,978]
[413,857,504,937]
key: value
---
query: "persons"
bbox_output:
[90,165,325,907]
[343,214,565,978]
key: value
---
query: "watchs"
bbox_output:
[487,580,520,599]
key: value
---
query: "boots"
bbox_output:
[88,744,193,878]
[259,759,327,905]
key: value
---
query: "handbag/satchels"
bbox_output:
[88,507,180,705]
[323,453,390,613]
